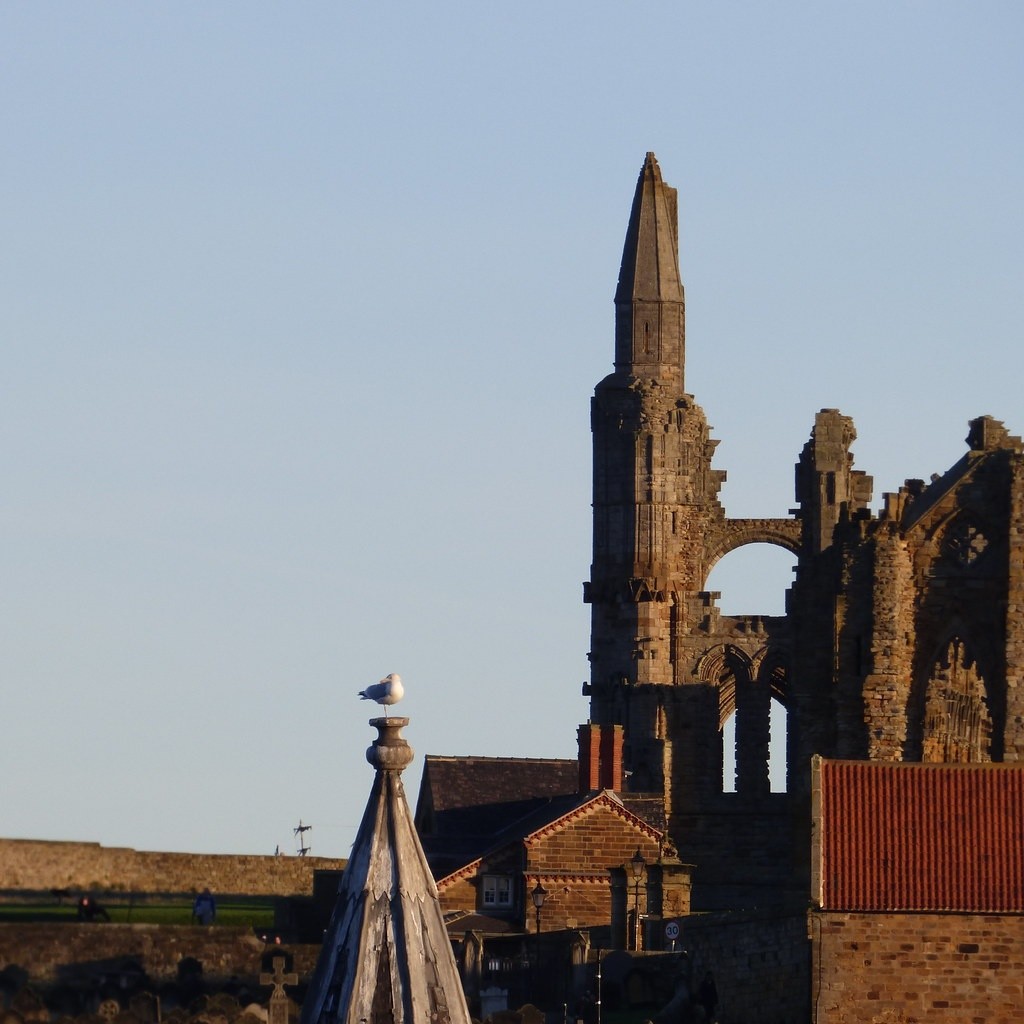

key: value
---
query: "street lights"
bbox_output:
[630,845,647,951]
[530,882,548,1005]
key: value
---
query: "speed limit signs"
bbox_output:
[666,921,679,940]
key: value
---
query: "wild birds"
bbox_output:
[360,672,403,719]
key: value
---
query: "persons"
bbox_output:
[698,971,718,1024]
[78,894,111,922]
[575,990,595,1024]
[194,888,215,926]
